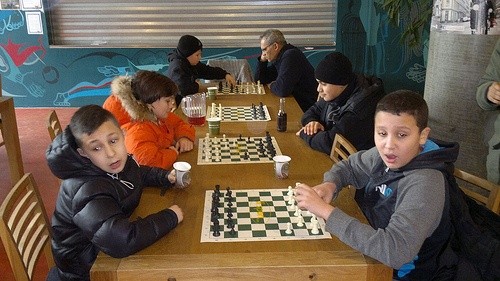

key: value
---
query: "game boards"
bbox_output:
[197,132,283,165]
[205,104,271,122]
[200,188,332,242]
[216,80,266,95]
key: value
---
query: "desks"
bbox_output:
[89,83,394,281]
[0,96,24,185]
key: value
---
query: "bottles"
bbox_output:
[277,98,287,132]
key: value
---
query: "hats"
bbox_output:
[178,35,203,58]
[314,53,353,86]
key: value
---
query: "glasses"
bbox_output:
[262,40,278,53]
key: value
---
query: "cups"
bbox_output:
[206,117,222,135]
[173,161,192,187]
[273,155,291,179]
[180,92,206,127]
[207,87,218,100]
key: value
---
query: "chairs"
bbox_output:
[329,133,357,198]
[205,59,254,82]
[47,110,62,142]
[454,167,500,214]
[0,172,56,281]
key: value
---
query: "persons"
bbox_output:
[102,70,196,169]
[45,104,184,281]
[292,90,481,281]
[475,40,500,184]
[295,54,388,162]
[252,30,320,113]
[160,35,236,109]
